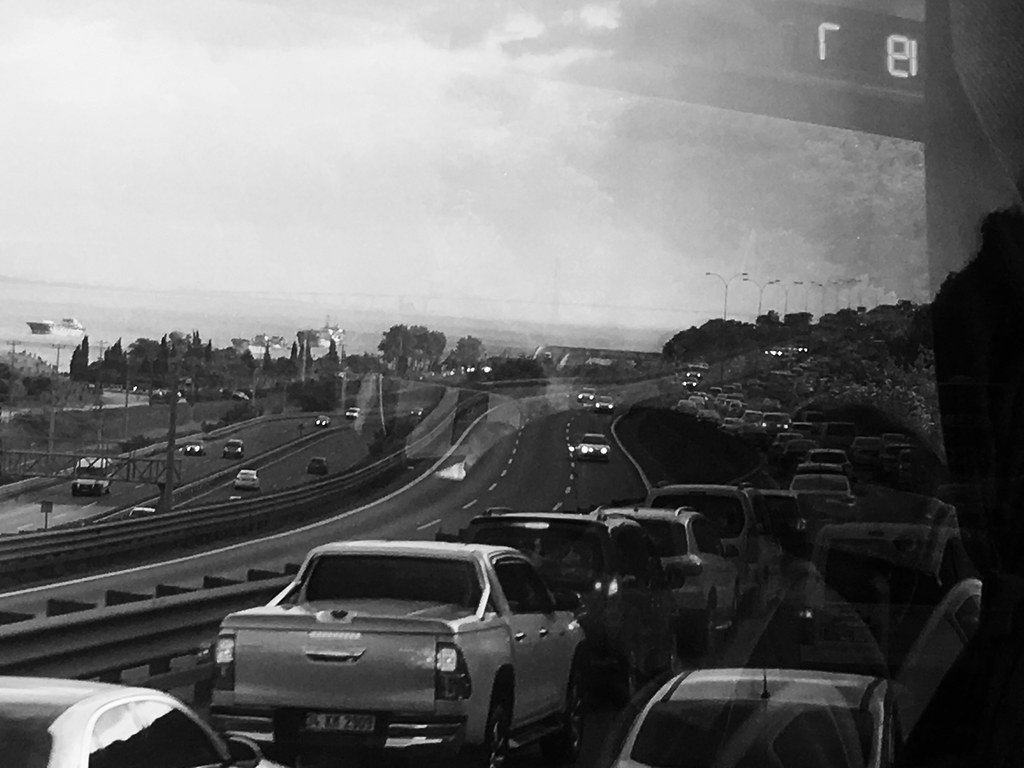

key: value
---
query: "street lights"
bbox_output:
[706,271,749,340]
[742,275,872,328]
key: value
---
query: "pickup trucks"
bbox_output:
[206,539,591,768]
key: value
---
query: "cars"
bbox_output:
[315,415,331,427]
[128,507,155,515]
[234,470,261,491]
[459,342,996,747]
[223,439,246,460]
[346,408,361,420]
[307,457,329,475]
[1,676,287,768]
[182,440,206,456]
[613,670,904,768]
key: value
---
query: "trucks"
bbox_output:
[70,457,114,497]
